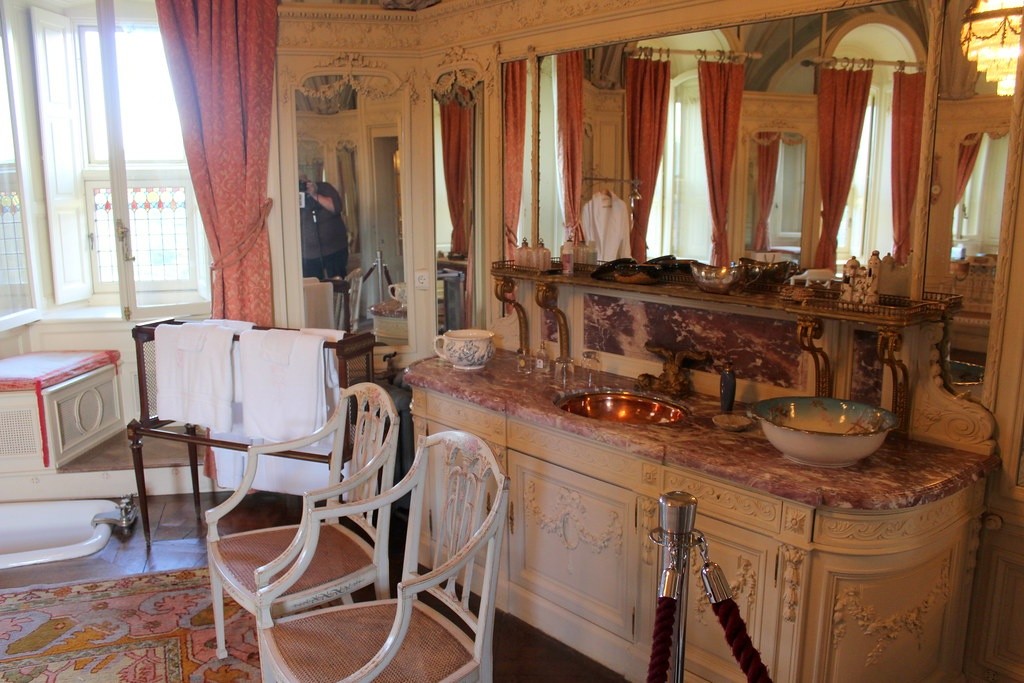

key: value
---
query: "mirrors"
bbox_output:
[500,0,949,299]
[422,45,493,337]
[731,89,823,266]
[283,53,416,356]
[927,97,1016,407]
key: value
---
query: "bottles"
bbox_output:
[720,363,737,414]
[560,235,598,265]
[561,247,574,275]
[513,237,551,271]
[534,342,551,373]
[513,348,532,374]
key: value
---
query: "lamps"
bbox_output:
[960,0,1024,97]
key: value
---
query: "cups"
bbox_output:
[553,357,575,387]
[580,351,600,388]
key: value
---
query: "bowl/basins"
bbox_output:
[644,255,695,272]
[738,257,794,285]
[751,395,901,468]
[433,329,496,370]
[388,283,407,308]
[612,263,663,285]
[688,258,762,295]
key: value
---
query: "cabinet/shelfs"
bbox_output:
[403,347,1002,683]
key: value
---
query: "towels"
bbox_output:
[153,323,233,434]
[184,318,343,490]
[236,327,332,445]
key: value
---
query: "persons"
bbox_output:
[299,172,350,282]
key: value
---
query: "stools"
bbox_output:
[1,349,128,476]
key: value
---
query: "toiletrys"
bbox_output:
[513,238,551,271]
[719,362,735,411]
[562,241,574,276]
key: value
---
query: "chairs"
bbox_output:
[333,266,364,332]
[256,430,512,683]
[203,381,400,660]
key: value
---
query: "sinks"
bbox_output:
[550,386,691,426]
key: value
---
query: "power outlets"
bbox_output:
[415,270,429,290]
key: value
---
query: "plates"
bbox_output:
[712,414,750,431]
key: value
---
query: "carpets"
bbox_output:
[0,566,335,683]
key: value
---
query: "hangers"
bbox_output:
[626,177,642,200]
[601,176,613,207]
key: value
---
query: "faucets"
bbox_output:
[638,343,710,397]
